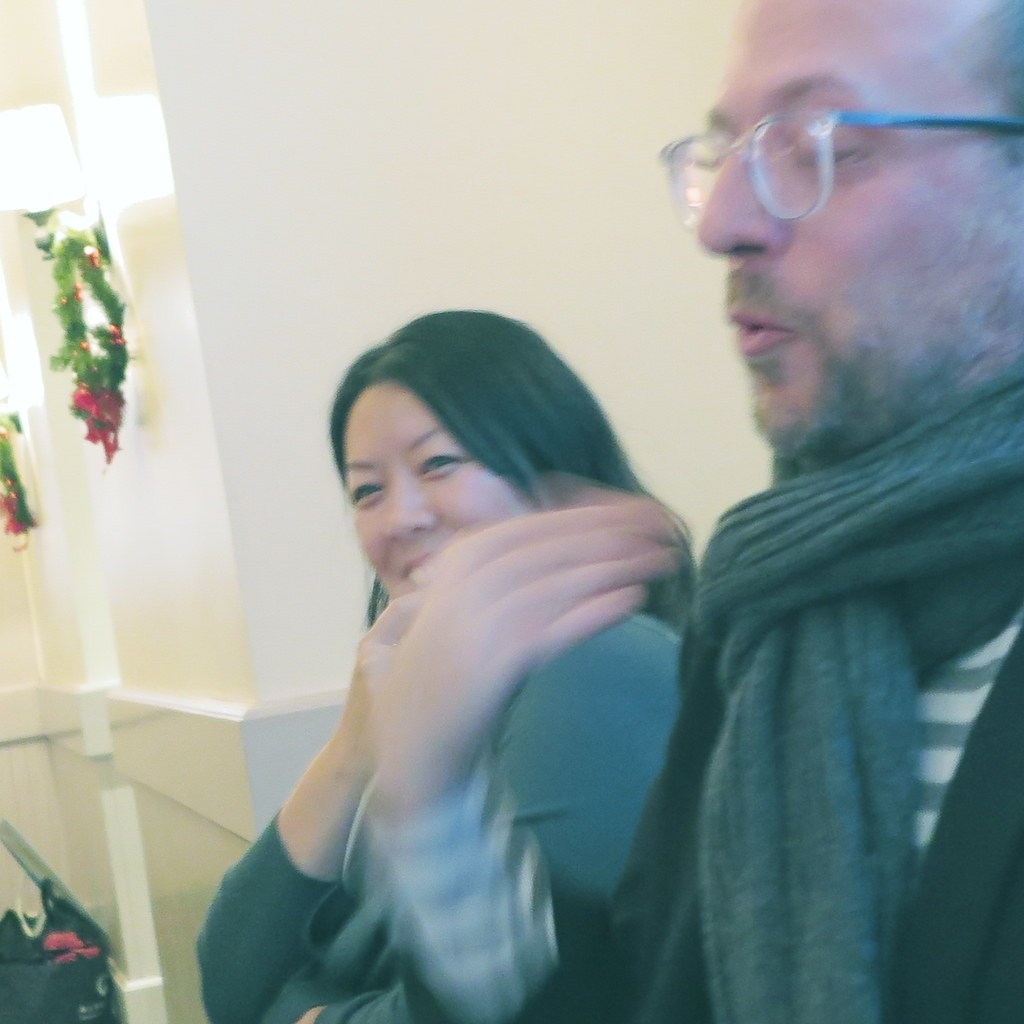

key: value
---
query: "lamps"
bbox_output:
[0,103,85,260]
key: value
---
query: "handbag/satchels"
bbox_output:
[0,878,126,1024]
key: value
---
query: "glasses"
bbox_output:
[661,107,1024,220]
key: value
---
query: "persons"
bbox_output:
[195,312,699,1023]
[365,0,1023,1023]
